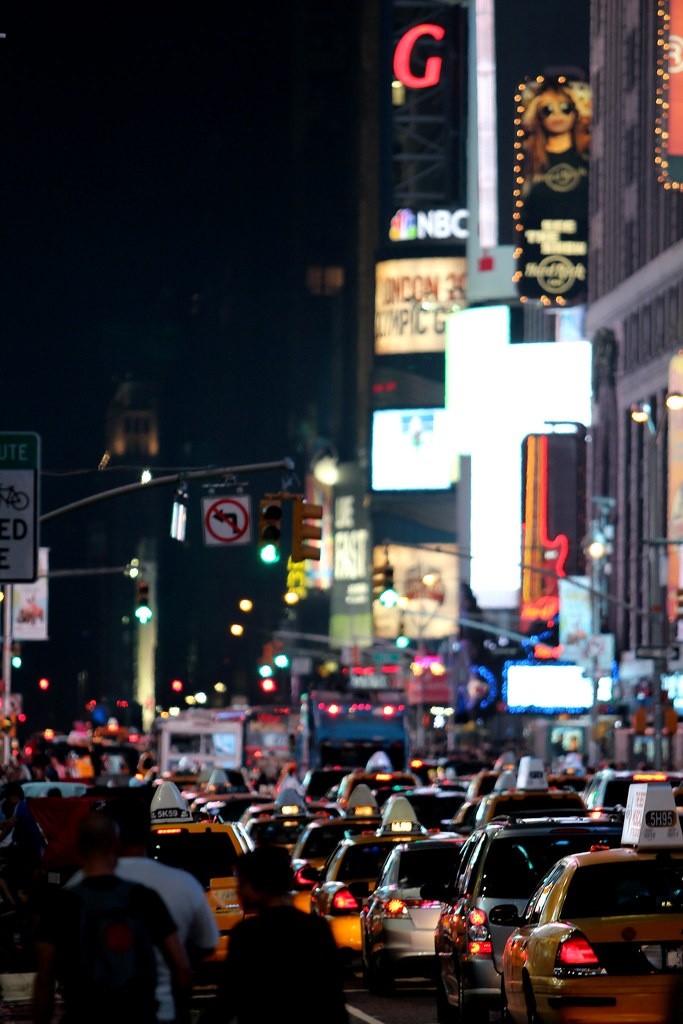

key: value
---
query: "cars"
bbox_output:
[488,775,683,1024]
[149,783,254,990]
[300,796,463,988]
[581,768,683,822]
[358,838,467,1008]
[40,739,593,913]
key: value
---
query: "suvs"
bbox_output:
[420,814,622,1024]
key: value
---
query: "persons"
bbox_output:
[581,752,677,775]
[62,792,221,1024]
[412,736,499,769]
[0,781,71,917]
[0,755,52,822]
[30,823,192,1024]
[196,845,354,1024]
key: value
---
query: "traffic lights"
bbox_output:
[259,498,281,563]
[134,583,152,623]
[373,565,394,600]
[291,501,322,562]
